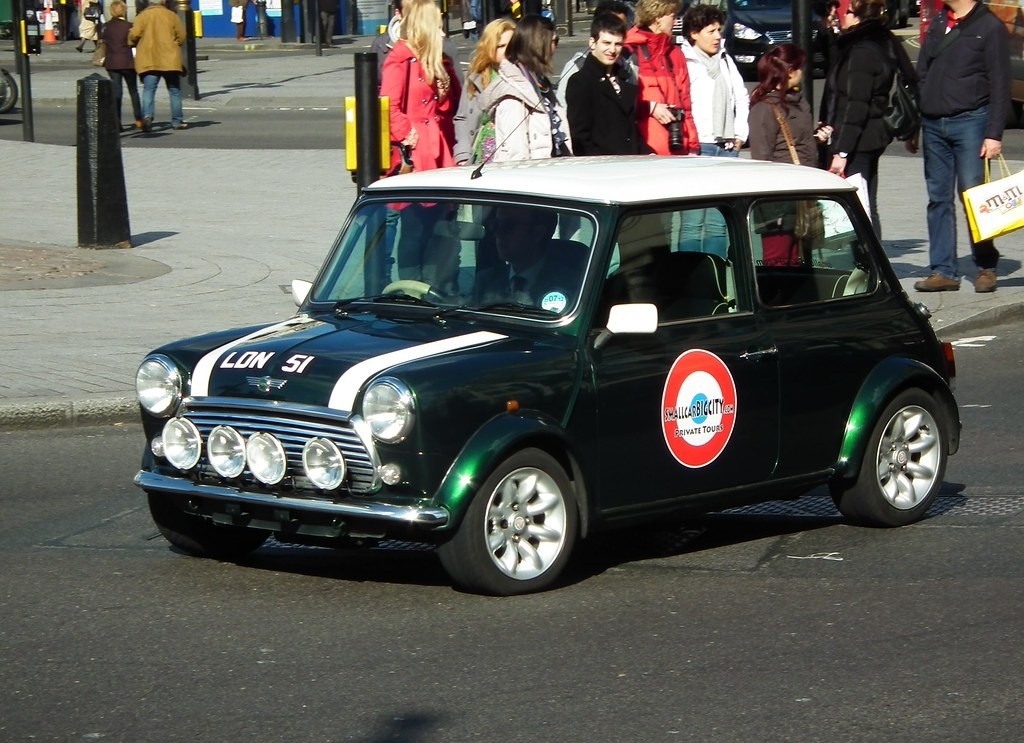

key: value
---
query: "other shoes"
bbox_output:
[142,117,153,132]
[239,36,249,41]
[75,46,83,52]
[136,120,143,128]
[172,122,189,130]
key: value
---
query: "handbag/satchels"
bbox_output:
[378,141,414,180]
[793,198,825,242]
[818,168,873,239]
[91,39,107,67]
[961,151,1024,245]
[230,5,243,24]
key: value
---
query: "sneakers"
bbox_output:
[913,273,962,292]
[974,269,998,293]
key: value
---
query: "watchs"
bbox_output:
[839,152,847,158]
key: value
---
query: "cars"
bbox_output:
[881,0,915,28]
[719,0,831,82]
[130,154,966,596]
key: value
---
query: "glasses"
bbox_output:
[552,36,560,47]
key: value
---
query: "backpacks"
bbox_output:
[845,24,922,142]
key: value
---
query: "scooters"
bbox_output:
[0,68,19,110]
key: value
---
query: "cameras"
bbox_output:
[664,107,685,150]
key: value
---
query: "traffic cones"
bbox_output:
[43,7,63,45]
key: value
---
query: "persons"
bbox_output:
[453,13,567,225]
[555,0,700,279]
[102,0,143,128]
[320,0,337,48]
[750,44,834,267]
[678,4,749,261]
[817,0,900,243]
[473,205,567,305]
[905,0,1012,293]
[128,0,188,131]
[76,0,101,52]
[370,0,463,295]
[229,0,249,41]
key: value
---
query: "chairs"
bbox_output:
[547,238,590,272]
[644,251,739,323]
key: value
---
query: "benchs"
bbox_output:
[755,264,868,304]
